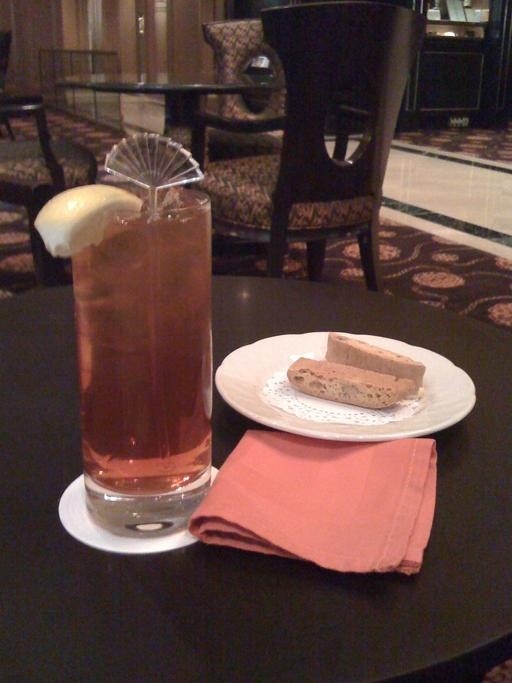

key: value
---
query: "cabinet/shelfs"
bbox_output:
[349,0,512,130]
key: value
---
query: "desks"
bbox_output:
[50,70,292,269]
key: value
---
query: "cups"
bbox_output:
[68,190,214,538]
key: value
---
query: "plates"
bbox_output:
[214,328,478,444]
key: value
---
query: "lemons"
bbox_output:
[33,183,144,260]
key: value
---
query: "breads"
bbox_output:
[287,332,426,410]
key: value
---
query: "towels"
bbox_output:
[185,417,441,587]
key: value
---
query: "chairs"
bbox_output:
[189,0,428,297]
[194,14,290,159]
[1,26,17,141]
[1,95,100,288]
